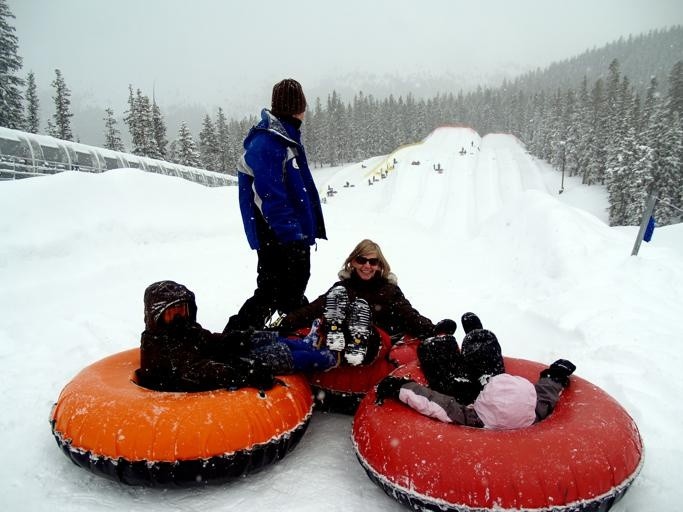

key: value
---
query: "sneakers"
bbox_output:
[323,285,375,367]
[303,317,327,353]
[433,319,457,335]
[320,346,341,374]
[461,311,484,334]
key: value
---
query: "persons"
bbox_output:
[222,79,327,335]
[134,280,342,390]
[286,238,436,367]
[328,139,474,196]
[375,311,578,430]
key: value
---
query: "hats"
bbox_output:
[272,77,307,117]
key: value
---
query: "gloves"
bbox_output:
[375,375,413,406]
[540,358,577,387]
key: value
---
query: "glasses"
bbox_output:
[355,255,380,266]
